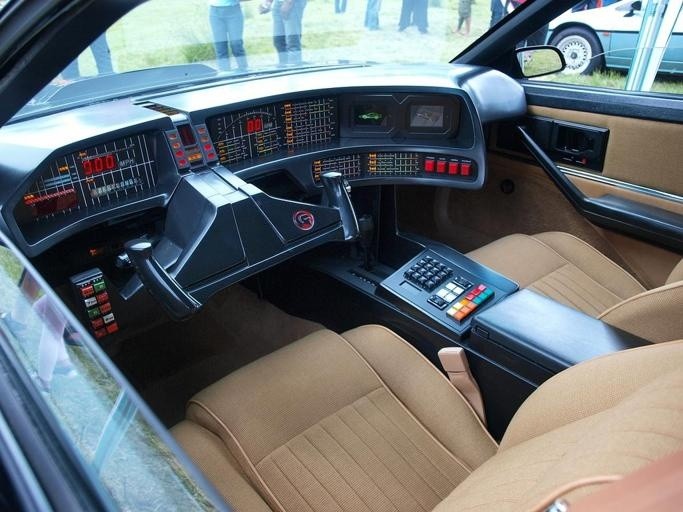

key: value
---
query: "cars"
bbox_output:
[523,0,683,75]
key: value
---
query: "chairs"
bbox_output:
[164,324,683,512]
[466,231,683,344]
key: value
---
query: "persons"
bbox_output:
[398,0,428,35]
[258,0,309,66]
[62,31,112,81]
[453,0,476,36]
[334,0,347,13]
[364,0,381,30]
[208,0,248,72]
[490,0,616,51]
